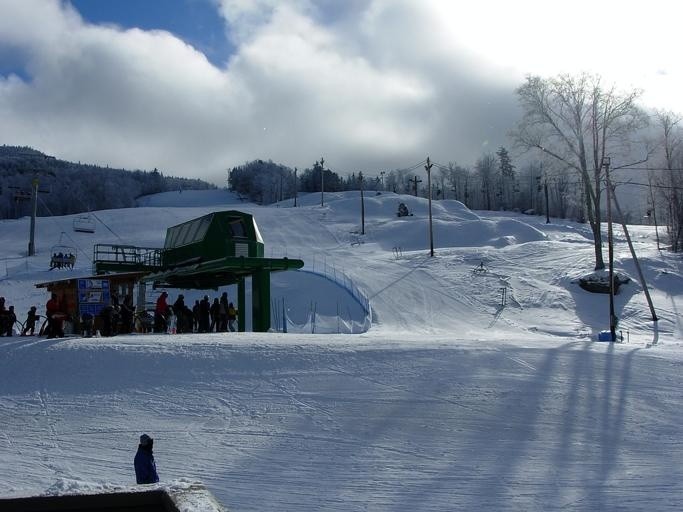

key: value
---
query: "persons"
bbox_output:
[50,252,75,270]
[0,291,238,339]
[134,434,159,483]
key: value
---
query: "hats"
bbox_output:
[139,434,153,446]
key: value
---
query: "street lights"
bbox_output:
[599,155,619,343]
